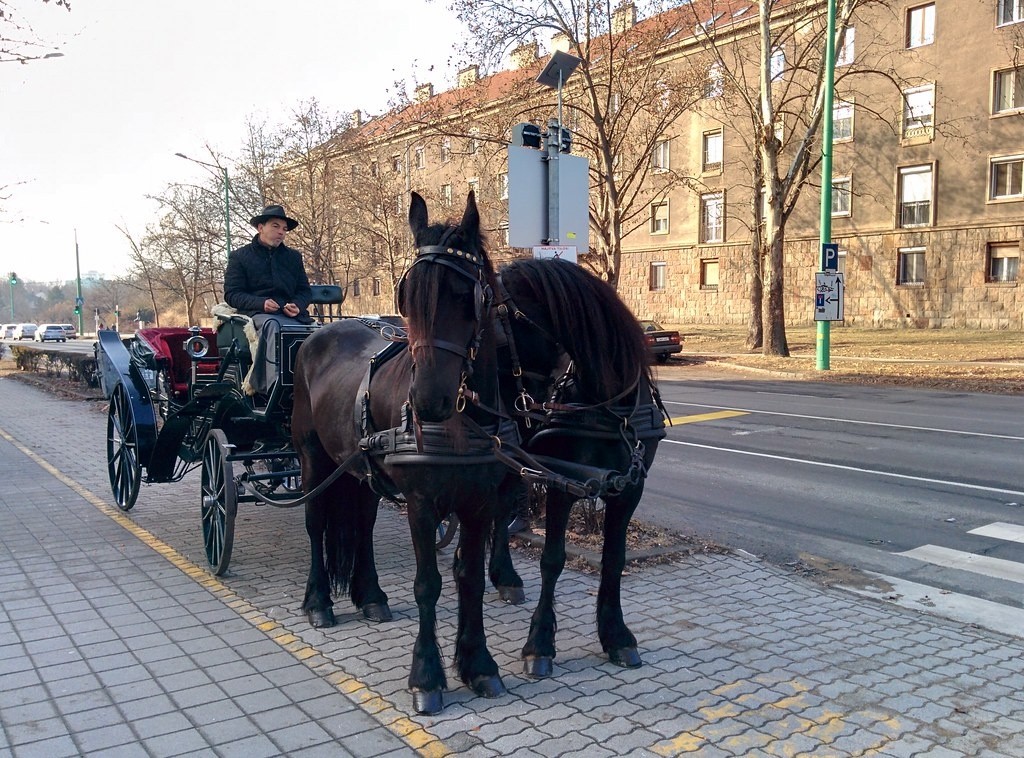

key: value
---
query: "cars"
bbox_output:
[0,323,18,339]
[636,318,684,363]
[12,323,38,340]
[60,324,77,339]
[33,324,67,343]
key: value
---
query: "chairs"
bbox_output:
[210,303,253,406]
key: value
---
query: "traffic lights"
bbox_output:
[114,311,121,317]
[74,306,79,314]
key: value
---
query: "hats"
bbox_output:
[250,205,299,232]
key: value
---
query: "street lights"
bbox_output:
[510,50,582,246]
[8,272,18,324]
[174,152,231,263]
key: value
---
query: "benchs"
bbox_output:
[157,327,226,404]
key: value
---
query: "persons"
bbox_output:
[223,206,315,400]
[99,322,116,331]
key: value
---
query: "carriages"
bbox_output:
[92,189,675,717]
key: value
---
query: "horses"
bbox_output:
[291,190,667,714]
